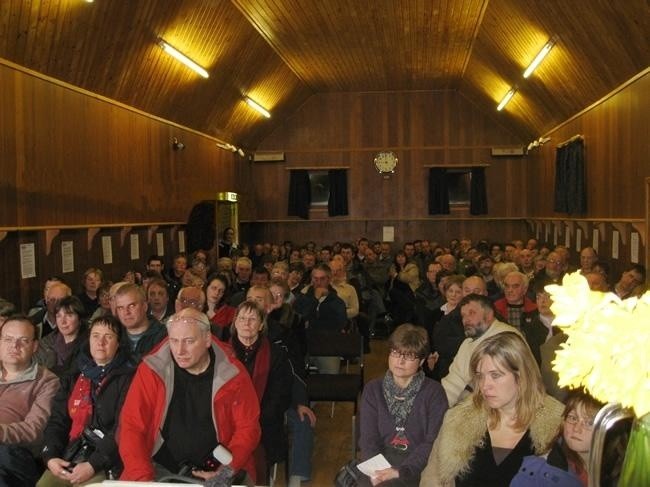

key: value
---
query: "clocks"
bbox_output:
[373,150,398,175]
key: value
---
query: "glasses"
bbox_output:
[166,317,207,328]
[565,412,593,431]
[390,350,419,360]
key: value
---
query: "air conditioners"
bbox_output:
[490,144,524,156]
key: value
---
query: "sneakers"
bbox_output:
[334,460,361,487]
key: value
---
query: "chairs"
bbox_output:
[587,404,635,487]
[296,331,365,459]
[252,445,270,486]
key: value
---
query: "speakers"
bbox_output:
[491,147,523,156]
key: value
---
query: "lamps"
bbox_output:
[522,35,560,79]
[496,83,519,112]
[243,96,271,119]
[156,37,210,79]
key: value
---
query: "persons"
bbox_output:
[1,228,646,486]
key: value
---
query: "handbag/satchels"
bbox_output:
[64,426,104,465]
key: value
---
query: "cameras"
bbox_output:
[64,425,104,471]
[177,443,233,482]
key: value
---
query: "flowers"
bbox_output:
[542,268,650,487]
